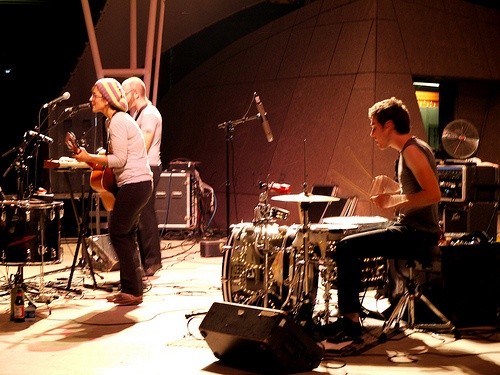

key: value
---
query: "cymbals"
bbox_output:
[270,192,340,203]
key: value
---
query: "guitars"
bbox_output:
[65,131,118,212]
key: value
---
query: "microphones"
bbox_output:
[28,130,54,145]
[64,103,90,112]
[255,94,274,143]
[44,91,70,109]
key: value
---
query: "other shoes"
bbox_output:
[329,315,363,342]
[106,294,144,305]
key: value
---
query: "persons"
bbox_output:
[73,78,154,306]
[122,77,163,276]
[324,99,441,344]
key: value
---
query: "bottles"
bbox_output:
[14,288,25,323]
[11,273,24,319]
[24,300,36,322]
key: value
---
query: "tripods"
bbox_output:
[242,191,291,308]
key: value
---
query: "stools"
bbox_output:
[379,256,461,343]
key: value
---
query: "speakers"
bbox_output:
[198,301,322,374]
[83,234,120,272]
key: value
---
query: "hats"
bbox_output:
[94,78,128,113]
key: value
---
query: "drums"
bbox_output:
[0,196,65,266]
[219,214,390,326]
